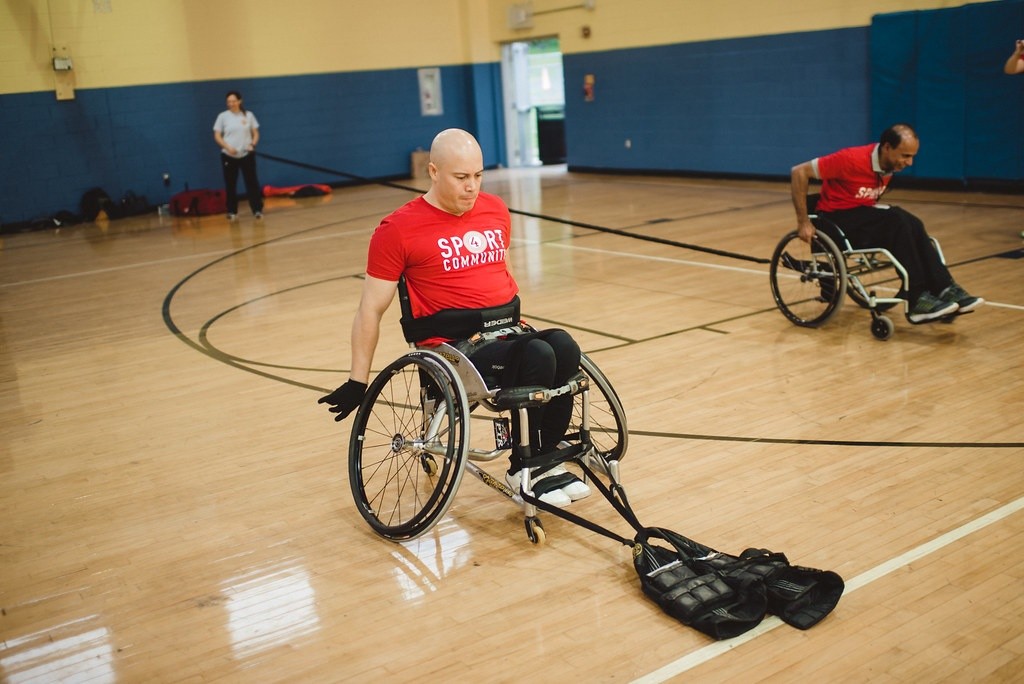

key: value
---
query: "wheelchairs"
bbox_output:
[769,192,976,341]
[347,274,629,547]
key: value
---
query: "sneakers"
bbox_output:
[909,291,960,323]
[546,463,592,500]
[504,466,571,509]
[938,283,985,312]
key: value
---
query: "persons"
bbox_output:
[318,126,592,511]
[1004,39,1024,237]
[790,123,985,324]
[212,91,264,223]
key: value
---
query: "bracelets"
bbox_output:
[249,143,255,148]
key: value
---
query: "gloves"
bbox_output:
[318,378,368,422]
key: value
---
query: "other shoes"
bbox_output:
[255,212,262,219]
[228,213,235,218]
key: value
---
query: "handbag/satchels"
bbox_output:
[170,190,229,217]
